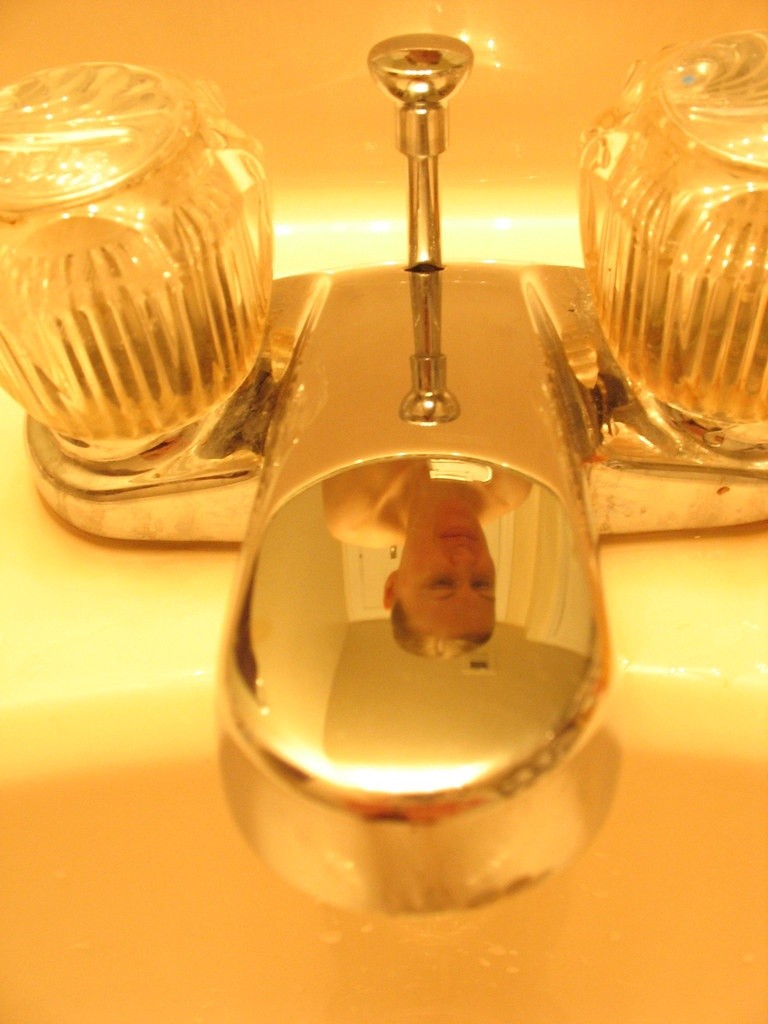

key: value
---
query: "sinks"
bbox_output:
[1,178,767,1024]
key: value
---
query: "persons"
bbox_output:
[323,461,531,662]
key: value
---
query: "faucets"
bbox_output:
[1,30,768,919]
